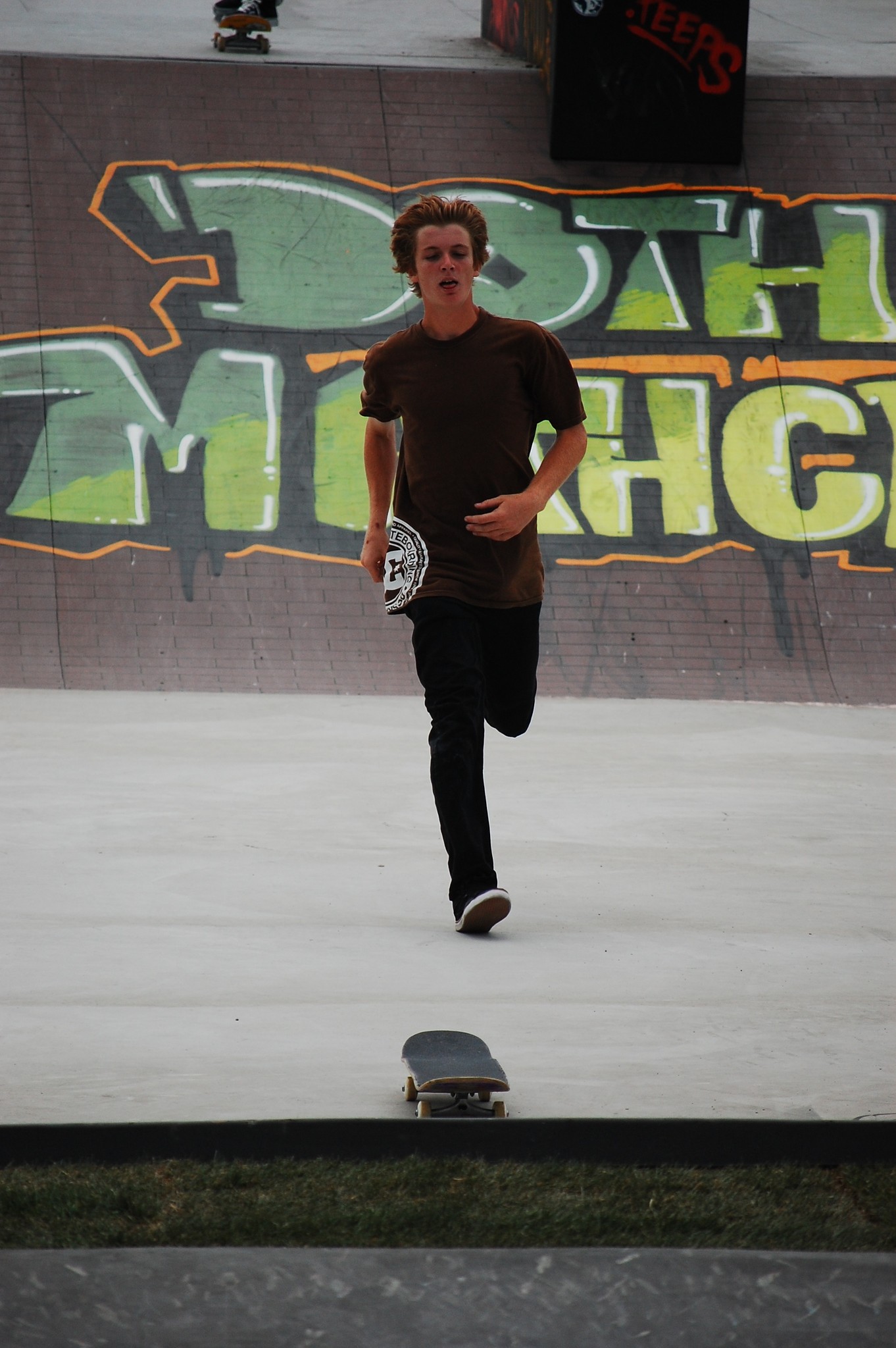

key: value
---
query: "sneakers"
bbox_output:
[221,0,278,28]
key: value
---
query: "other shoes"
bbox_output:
[453,888,511,934]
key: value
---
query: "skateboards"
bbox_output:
[400,1030,510,1118]
[213,14,271,55]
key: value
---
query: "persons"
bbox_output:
[360,196,590,935]
[213,0,281,29]
